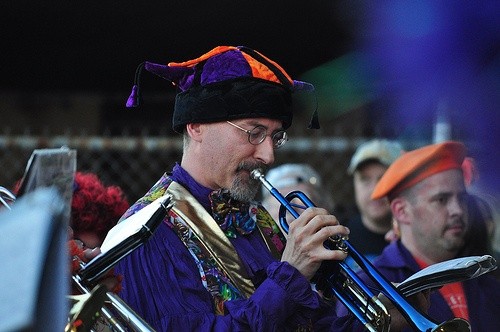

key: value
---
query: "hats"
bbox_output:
[371,140,466,204]
[261,163,322,199]
[347,138,406,177]
[125,46,321,131]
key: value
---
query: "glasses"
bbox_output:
[227,121,289,150]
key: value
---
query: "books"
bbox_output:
[394,254,498,299]
[78,194,176,286]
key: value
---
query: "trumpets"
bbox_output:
[249,168,472,332]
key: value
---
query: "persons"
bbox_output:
[336,140,500,332]
[115,45,444,332]
[68,223,119,299]
[342,139,402,257]
[261,163,322,240]
[14,170,129,248]
